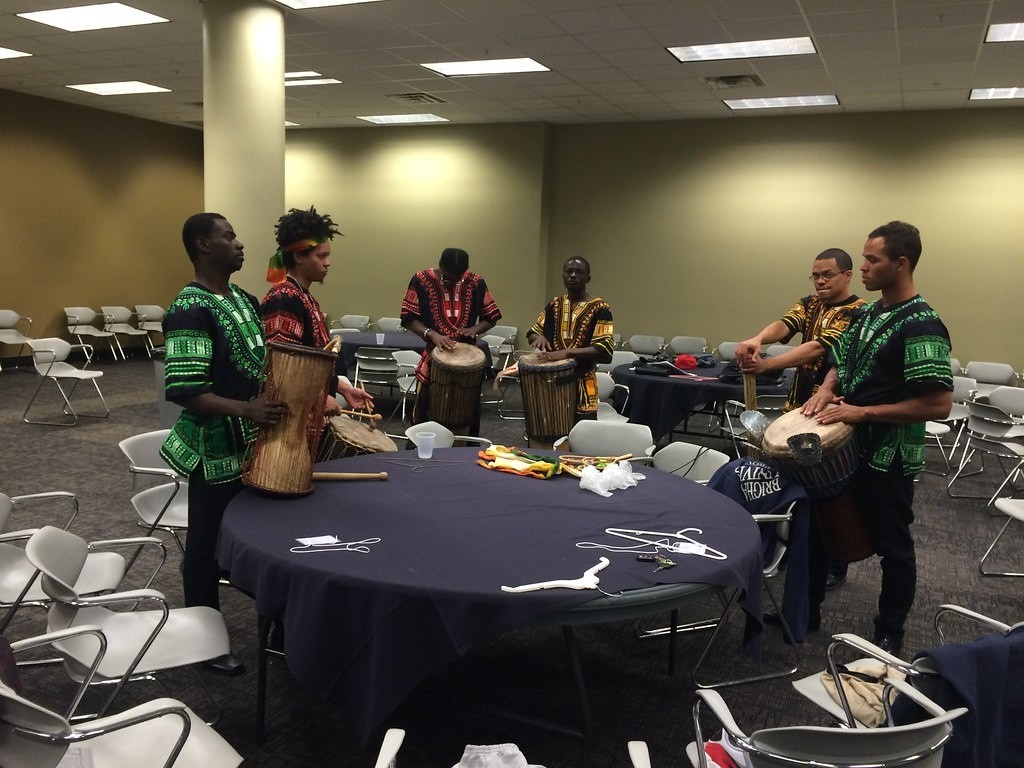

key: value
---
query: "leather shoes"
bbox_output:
[196,655,244,674]
[826,573,848,589]
[764,609,821,630]
[871,631,902,656]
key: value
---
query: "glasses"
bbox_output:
[439,271,463,283]
[809,269,850,281]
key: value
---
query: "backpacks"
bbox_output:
[716,353,786,387]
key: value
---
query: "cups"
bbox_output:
[376,333,385,345]
[416,432,436,459]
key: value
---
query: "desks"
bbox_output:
[213,448,763,768]
[328,332,494,396]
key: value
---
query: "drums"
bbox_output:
[427,341,487,435]
[761,402,877,562]
[242,338,338,496]
[518,353,579,450]
[315,411,398,464]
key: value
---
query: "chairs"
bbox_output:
[375,727,651,768]
[0,306,244,768]
[322,312,545,448]
[554,334,1024,768]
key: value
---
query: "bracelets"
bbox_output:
[423,329,429,338]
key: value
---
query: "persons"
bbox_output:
[260,206,376,454]
[162,212,290,676]
[734,249,866,589]
[527,256,615,423]
[400,248,502,451]
[761,219,954,658]
